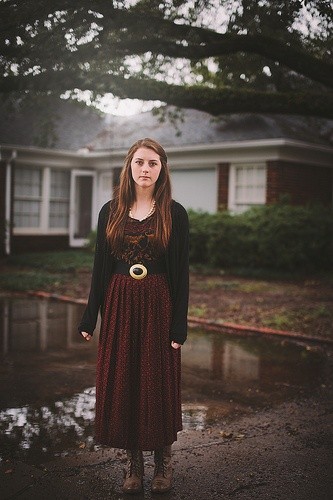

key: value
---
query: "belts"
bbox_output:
[112,261,167,280]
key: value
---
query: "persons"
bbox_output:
[79,138,191,495]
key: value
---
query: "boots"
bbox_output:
[150,444,173,494]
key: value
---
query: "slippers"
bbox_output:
[123,449,145,494]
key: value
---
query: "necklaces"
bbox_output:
[129,198,156,222]
